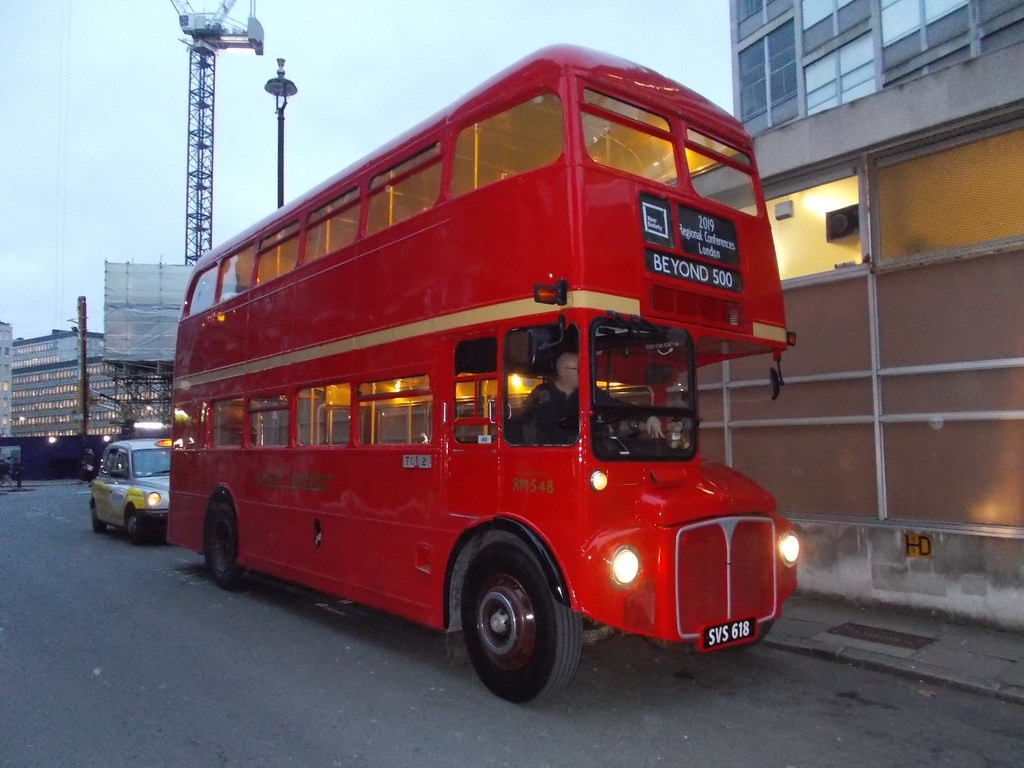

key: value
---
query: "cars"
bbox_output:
[88,439,171,546]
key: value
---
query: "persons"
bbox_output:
[86,448,100,487]
[0,457,17,489]
[522,353,666,445]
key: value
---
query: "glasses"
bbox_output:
[565,366,578,373]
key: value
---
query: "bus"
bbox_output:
[165,41,798,709]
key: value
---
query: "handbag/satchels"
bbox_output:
[86,464,93,471]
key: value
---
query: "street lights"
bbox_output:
[263,56,298,208]
